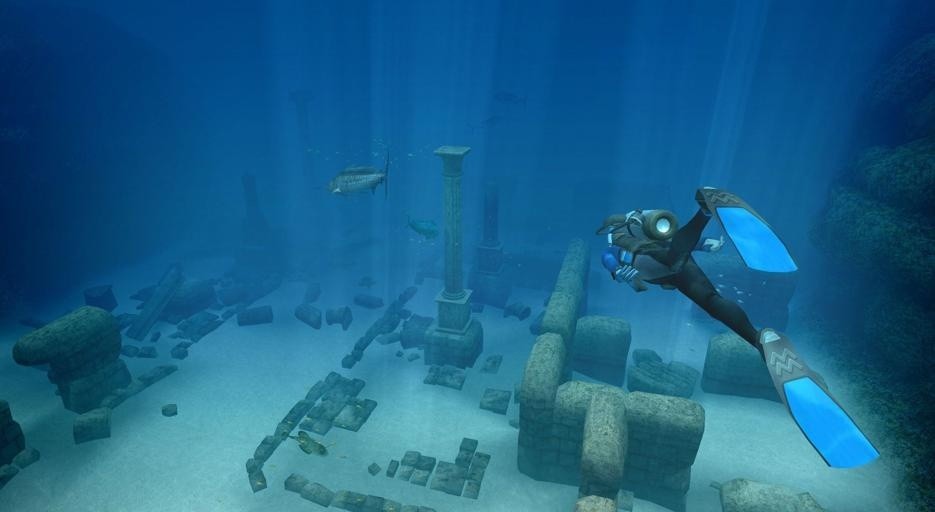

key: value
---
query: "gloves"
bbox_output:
[701,235,725,252]
[615,265,640,283]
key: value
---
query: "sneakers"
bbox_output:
[695,189,709,209]
[755,327,768,363]
